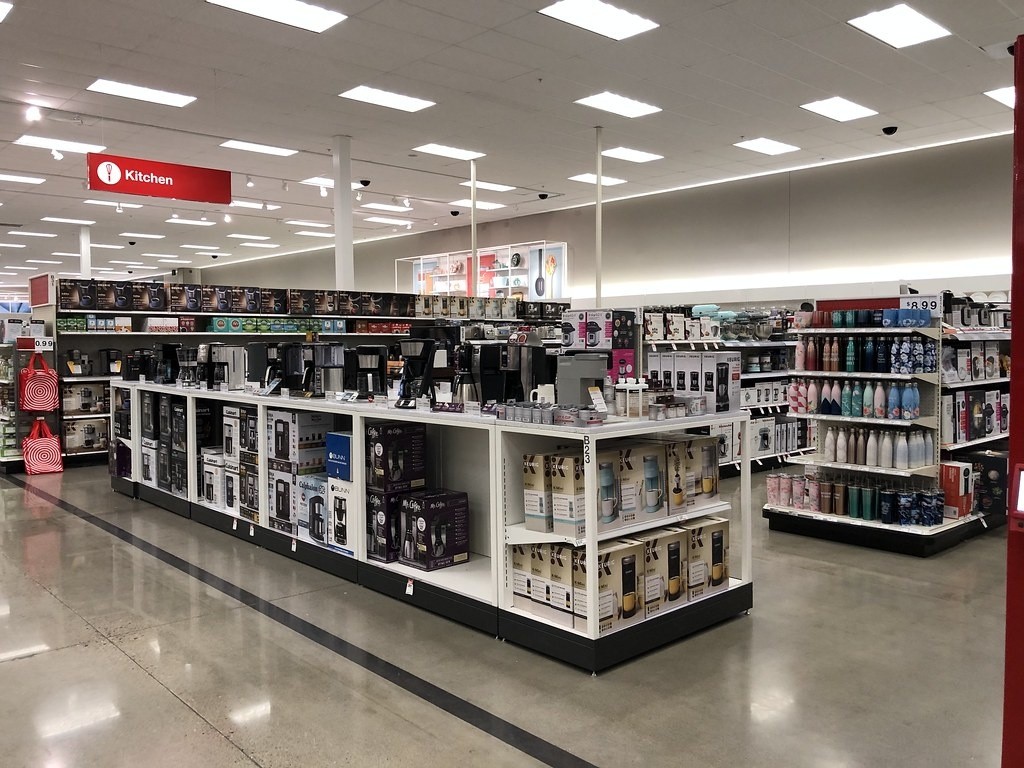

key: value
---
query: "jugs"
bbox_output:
[530,384,555,405]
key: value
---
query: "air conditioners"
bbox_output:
[262,200,268,211]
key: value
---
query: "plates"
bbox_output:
[512,253,521,268]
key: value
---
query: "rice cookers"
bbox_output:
[942,290,1011,330]
[562,322,603,347]
[100,347,153,379]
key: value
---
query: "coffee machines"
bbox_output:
[205,417,351,546]
[77,283,466,317]
[643,456,661,513]
[716,362,729,412]
[712,530,724,586]
[667,541,682,601]
[556,352,608,407]
[153,341,388,406]
[974,356,1009,433]
[396,339,546,408]
[650,370,714,391]
[700,447,717,499]
[598,462,618,524]
[621,555,636,618]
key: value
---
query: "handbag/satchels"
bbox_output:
[21,420,63,475]
[18,353,60,412]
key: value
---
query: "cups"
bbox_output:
[648,403,685,420]
[812,309,932,328]
[96,402,104,414]
[622,563,730,611]
[766,472,945,526]
[603,475,718,517]
[100,439,107,449]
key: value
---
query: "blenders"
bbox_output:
[757,387,787,402]
[77,388,96,448]
[758,428,772,452]
[716,434,728,457]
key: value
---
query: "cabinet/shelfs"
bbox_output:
[0,273,573,469]
[562,309,817,482]
[108,337,753,677]
[765,292,1011,559]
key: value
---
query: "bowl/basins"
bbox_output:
[93,444,101,450]
[794,311,812,329]
[90,406,98,413]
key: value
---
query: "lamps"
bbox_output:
[116,202,124,212]
[246,175,254,187]
[392,196,400,205]
[281,179,289,191]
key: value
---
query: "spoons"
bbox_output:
[534,249,545,297]
[545,255,558,300]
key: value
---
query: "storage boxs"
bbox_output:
[113,390,470,571]
[56,278,571,334]
[939,342,1011,520]
[62,384,108,453]
[0,317,45,456]
[512,352,818,637]
[561,312,720,350]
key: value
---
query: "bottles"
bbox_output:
[795,334,937,374]
[605,375,648,418]
[823,425,934,469]
[788,377,920,421]
